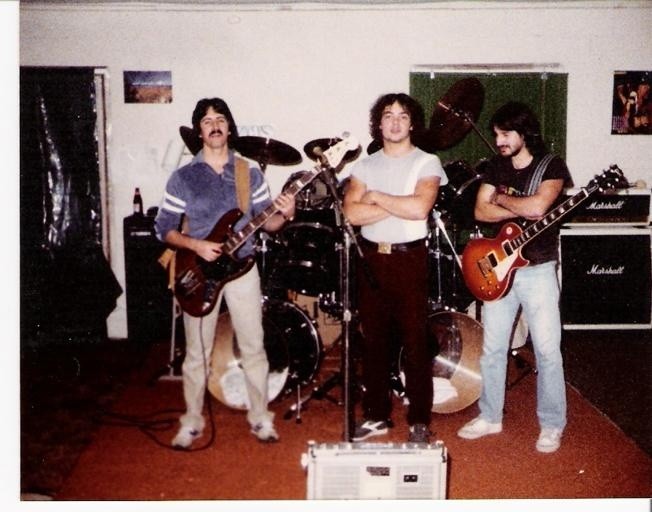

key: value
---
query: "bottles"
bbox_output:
[133,187,144,217]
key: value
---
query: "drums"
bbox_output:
[432,231,475,310]
[206,297,322,410]
[391,300,484,415]
[428,161,482,231]
[266,209,340,296]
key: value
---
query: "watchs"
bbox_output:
[283,212,295,226]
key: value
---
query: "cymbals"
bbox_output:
[233,137,302,166]
[367,129,430,154]
[179,125,204,155]
[430,78,484,152]
[302,138,363,162]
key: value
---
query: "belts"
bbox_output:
[362,238,423,255]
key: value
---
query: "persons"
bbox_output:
[340,94,449,448]
[457,106,571,456]
[155,98,298,450]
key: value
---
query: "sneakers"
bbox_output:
[171,426,203,448]
[352,420,388,443]
[249,421,279,443]
[407,423,430,443]
[458,416,502,439]
[536,427,565,453]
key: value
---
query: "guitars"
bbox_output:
[462,164,629,302]
[174,133,359,316]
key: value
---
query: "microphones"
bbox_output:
[306,139,332,172]
